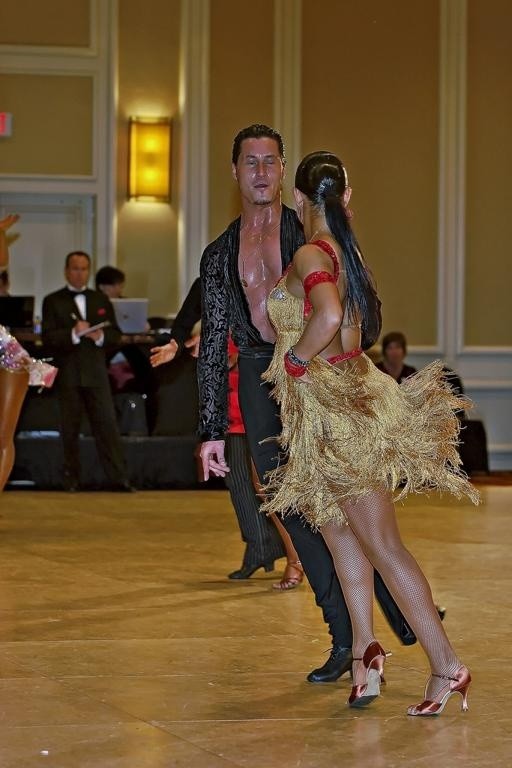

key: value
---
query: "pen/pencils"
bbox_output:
[71,313,80,322]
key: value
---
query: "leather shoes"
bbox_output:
[228,563,274,580]
[307,645,352,684]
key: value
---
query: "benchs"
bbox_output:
[454,418,490,475]
[12,430,200,487]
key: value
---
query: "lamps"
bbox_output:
[123,113,171,203]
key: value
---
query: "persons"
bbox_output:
[41,253,138,494]
[0,215,59,495]
[96,267,165,334]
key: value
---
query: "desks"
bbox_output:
[8,331,198,486]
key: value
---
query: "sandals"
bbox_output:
[271,557,306,591]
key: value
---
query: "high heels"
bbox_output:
[348,642,387,707]
[408,663,473,716]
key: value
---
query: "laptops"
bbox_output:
[110,301,148,334]
[0,297,34,329]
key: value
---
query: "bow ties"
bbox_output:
[70,289,89,299]
